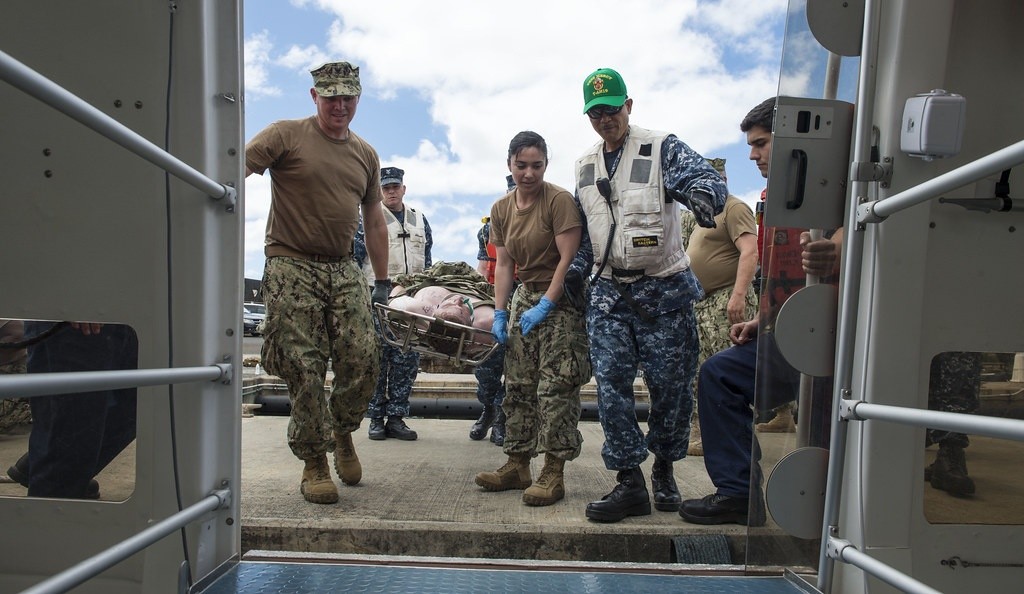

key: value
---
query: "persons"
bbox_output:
[0,319,137,499]
[468,131,592,507]
[354,168,433,440]
[386,261,508,355]
[676,93,983,530]
[244,61,389,503]
[564,67,759,524]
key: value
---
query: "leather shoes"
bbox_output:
[490,423,505,445]
[679,492,766,527]
[651,457,683,511]
[586,467,652,520]
[368,418,385,438]
[470,406,495,439]
[384,418,418,441]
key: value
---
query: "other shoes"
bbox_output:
[7,453,100,499]
[686,439,703,455]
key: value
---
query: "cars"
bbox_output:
[243,302,266,337]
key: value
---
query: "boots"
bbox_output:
[523,453,564,506]
[300,454,338,504]
[474,453,532,491]
[331,430,362,486]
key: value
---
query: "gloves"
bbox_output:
[492,309,507,344]
[565,269,583,297]
[519,296,557,336]
[372,280,390,308]
[667,188,717,228]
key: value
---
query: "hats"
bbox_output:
[705,158,726,171]
[309,61,362,97]
[583,68,627,113]
[381,167,404,187]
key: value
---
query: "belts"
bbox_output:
[265,243,350,261]
[522,281,552,292]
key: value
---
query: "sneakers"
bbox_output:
[924,447,975,494]
[756,415,796,433]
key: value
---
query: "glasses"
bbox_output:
[586,99,628,119]
